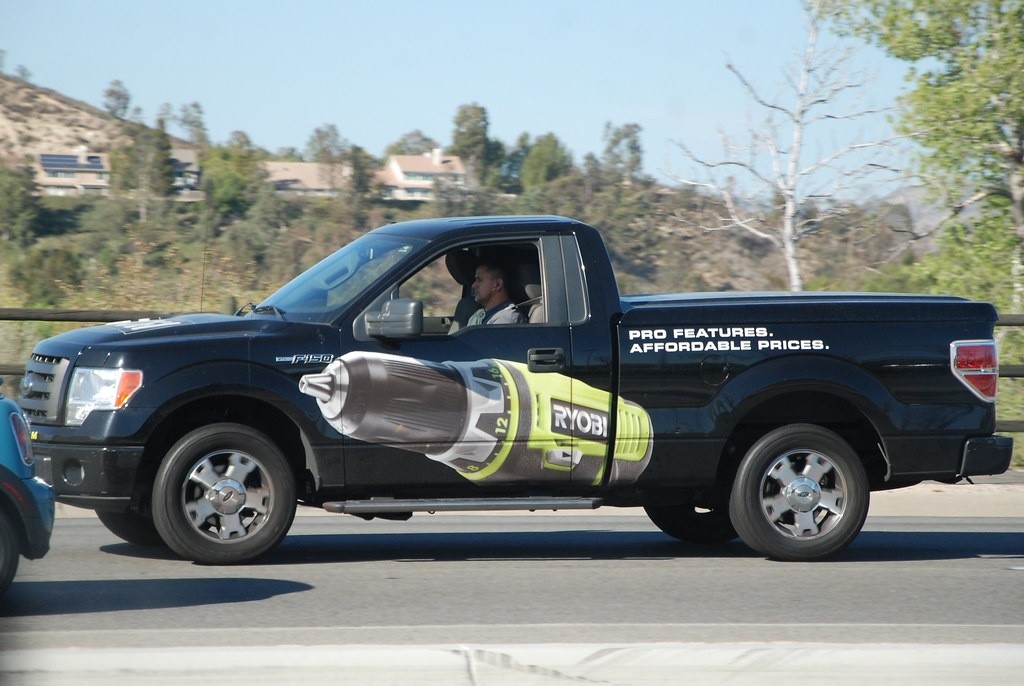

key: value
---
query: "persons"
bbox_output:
[467,256,526,325]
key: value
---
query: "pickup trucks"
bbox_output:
[14,215,1014,562]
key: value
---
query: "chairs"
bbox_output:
[445,249,544,335]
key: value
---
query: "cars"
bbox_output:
[1,393,56,596]
